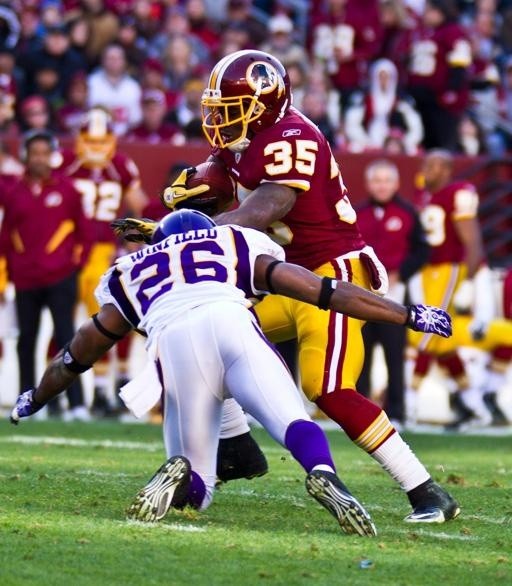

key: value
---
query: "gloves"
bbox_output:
[109,213,160,245]
[403,302,454,339]
[8,385,40,427]
[159,165,213,212]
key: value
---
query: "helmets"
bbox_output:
[149,206,218,248]
[205,46,294,137]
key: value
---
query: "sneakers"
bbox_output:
[125,452,191,527]
[302,468,379,540]
[214,430,270,489]
[399,480,462,526]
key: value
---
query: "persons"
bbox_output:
[2,2,511,425]
[115,50,461,525]
[9,207,452,536]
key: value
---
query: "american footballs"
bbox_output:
[175,161,234,216]
